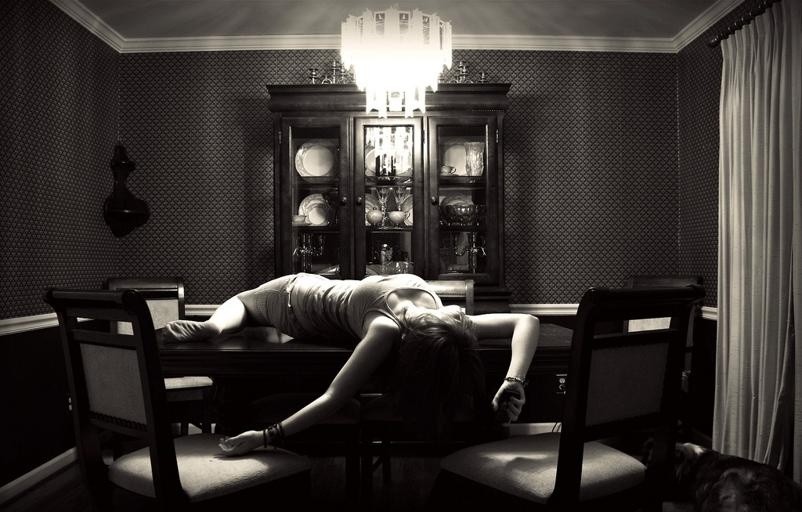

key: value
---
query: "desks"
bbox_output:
[114,317,598,511]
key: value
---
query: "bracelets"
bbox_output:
[263,420,284,448]
[503,374,525,384]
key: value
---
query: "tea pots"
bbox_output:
[384,204,411,230]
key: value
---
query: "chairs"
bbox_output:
[364,278,473,512]
[42,289,315,512]
[234,271,359,503]
[634,273,705,445]
[442,285,699,510]
[108,279,216,437]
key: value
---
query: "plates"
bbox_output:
[301,175,337,185]
[294,138,338,177]
[443,144,468,177]
[298,193,330,226]
[291,222,312,227]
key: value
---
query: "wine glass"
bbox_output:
[375,186,408,230]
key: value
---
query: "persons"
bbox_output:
[166,272,540,458]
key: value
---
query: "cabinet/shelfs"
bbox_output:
[265,82,515,300]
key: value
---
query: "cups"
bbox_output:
[465,142,486,176]
[441,166,457,174]
[292,215,306,223]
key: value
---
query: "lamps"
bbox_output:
[101,142,150,239]
[339,1,454,119]
